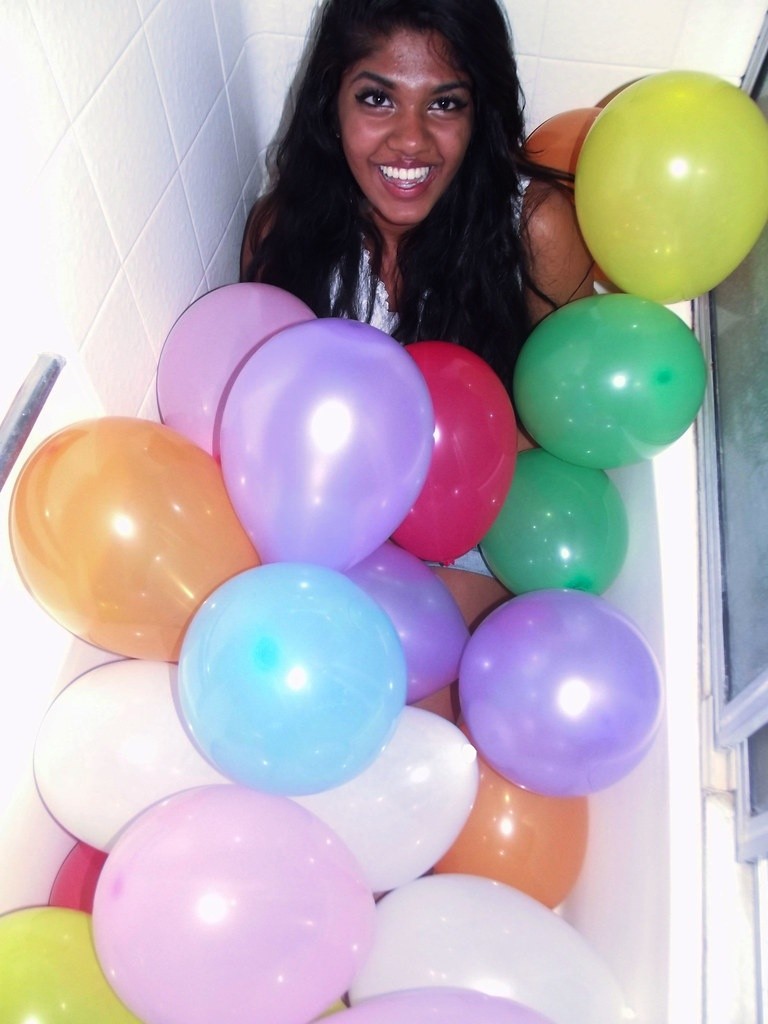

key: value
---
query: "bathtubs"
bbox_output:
[85,183,703,1024]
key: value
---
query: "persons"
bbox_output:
[238,1,598,721]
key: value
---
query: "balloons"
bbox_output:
[94,785,377,1023]
[316,985,554,1024]
[340,540,472,706]
[512,293,709,471]
[433,725,589,909]
[573,67,768,309]
[479,447,629,597]
[178,563,410,796]
[525,106,605,186]
[346,872,635,1024]
[290,706,481,894]
[1,906,140,1024]
[33,657,229,856]
[220,317,435,573]
[157,282,316,460]
[49,841,109,915]
[459,589,662,797]
[10,416,263,663]
[389,341,517,567]
